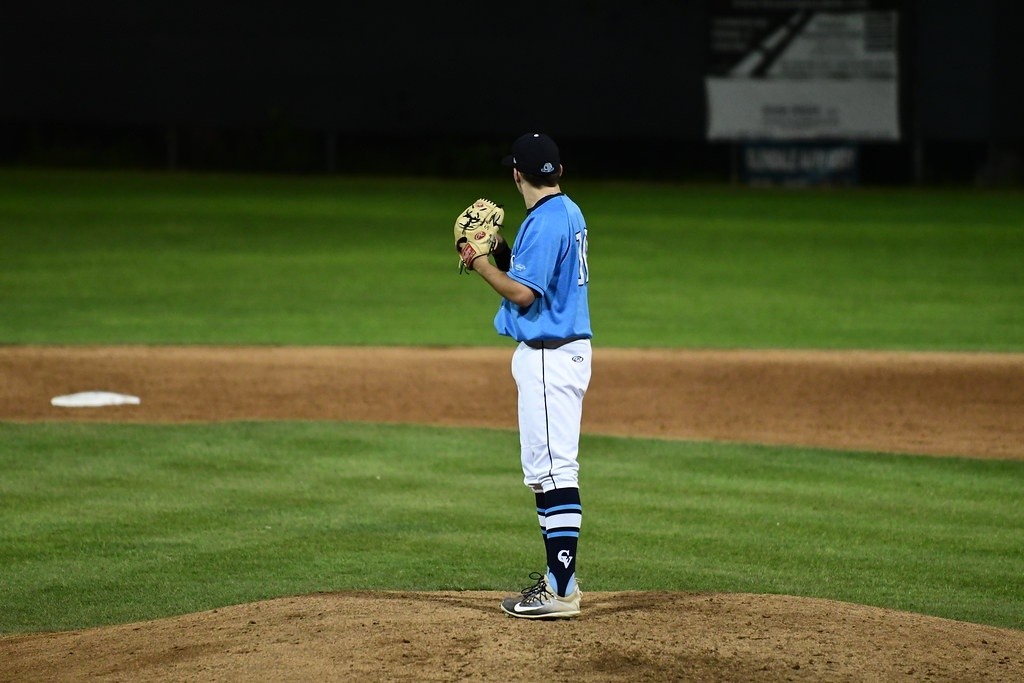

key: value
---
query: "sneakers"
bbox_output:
[502,573,582,618]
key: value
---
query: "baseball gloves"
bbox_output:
[454,197,505,275]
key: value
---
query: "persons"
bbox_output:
[454,130,594,619]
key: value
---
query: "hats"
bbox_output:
[502,133,559,175]
